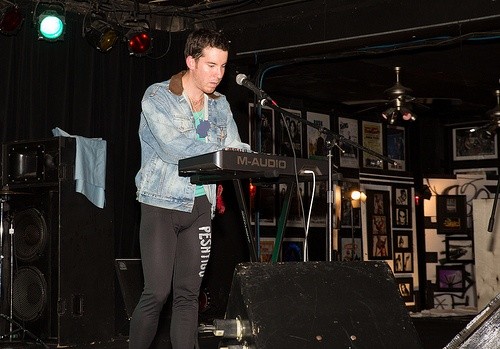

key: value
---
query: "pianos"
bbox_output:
[178,147,334,264]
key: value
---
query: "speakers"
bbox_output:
[209,260,424,349]
[0,135,116,348]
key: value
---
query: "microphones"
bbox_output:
[235,73,274,102]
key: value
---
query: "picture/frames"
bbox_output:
[248,102,416,301]
[436,197,468,233]
[435,264,467,294]
[452,121,499,160]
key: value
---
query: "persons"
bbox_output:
[396,189,414,302]
[280,187,286,202]
[129,29,251,349]
[374,192,389,257]
[262,113,268,139]
[285,117,301,156]
[315,130,328,156]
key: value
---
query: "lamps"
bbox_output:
[380,102,418,123]
[0,0,166,54]
[445,80,500,136]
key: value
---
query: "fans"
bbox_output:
[341,66,462,105]
